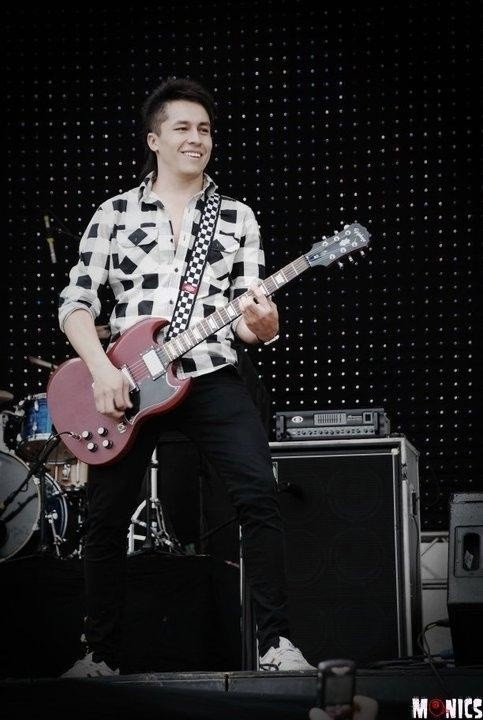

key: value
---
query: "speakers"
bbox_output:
[447,492,483,669]
[239,437,423,672]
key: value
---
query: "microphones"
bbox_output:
[44,216,57,264]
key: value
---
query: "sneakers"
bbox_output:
[58,652,121,678]
[258,636,317,671]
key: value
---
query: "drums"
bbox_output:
[18,393,79,465]
[47,461,87,493]
[0,450,68,566]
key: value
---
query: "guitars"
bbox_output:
[47,222,371,463]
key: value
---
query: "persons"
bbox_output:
[54,76,322,678]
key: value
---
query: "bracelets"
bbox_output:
[257,330,280,347]
